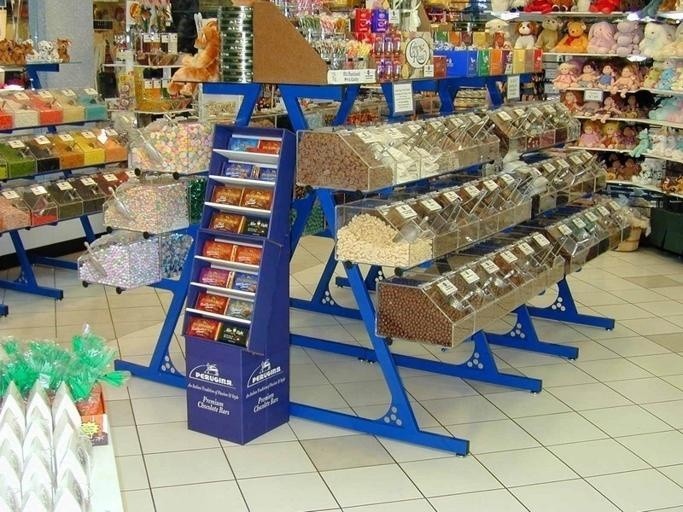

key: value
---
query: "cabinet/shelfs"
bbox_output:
[0,41,128,316]
[93,0,505,95]
[505,10,683,209]
[108,66,635,457]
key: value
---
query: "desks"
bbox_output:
[89,414,123,512]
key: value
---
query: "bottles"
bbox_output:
[344,57,363,69]
[373,30,401,82]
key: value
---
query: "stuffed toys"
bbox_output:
[163,17,219,97]
[483,1,682,198]
[0,36,71,66]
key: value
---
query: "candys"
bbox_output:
[79,124,215,291]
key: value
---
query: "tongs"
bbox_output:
[403,110,599,265]
